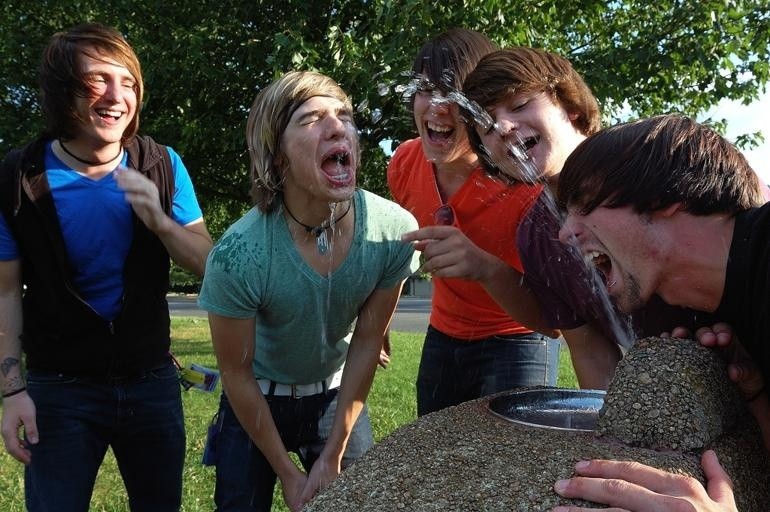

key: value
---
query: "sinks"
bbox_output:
[486,387,609,434]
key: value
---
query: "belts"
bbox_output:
[253,369,344,400]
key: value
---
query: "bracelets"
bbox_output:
[1,386,27,398]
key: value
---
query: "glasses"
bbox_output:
[431,203,460,230]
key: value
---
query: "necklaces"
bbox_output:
[278,195,354,258]
[53,135,124,167]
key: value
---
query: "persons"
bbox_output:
[552,113,770,512]
[196,69,422,511]
[386,26,563,420]
[378,326,391,370]
[458,44,702,391]
[0,24,217,511]
